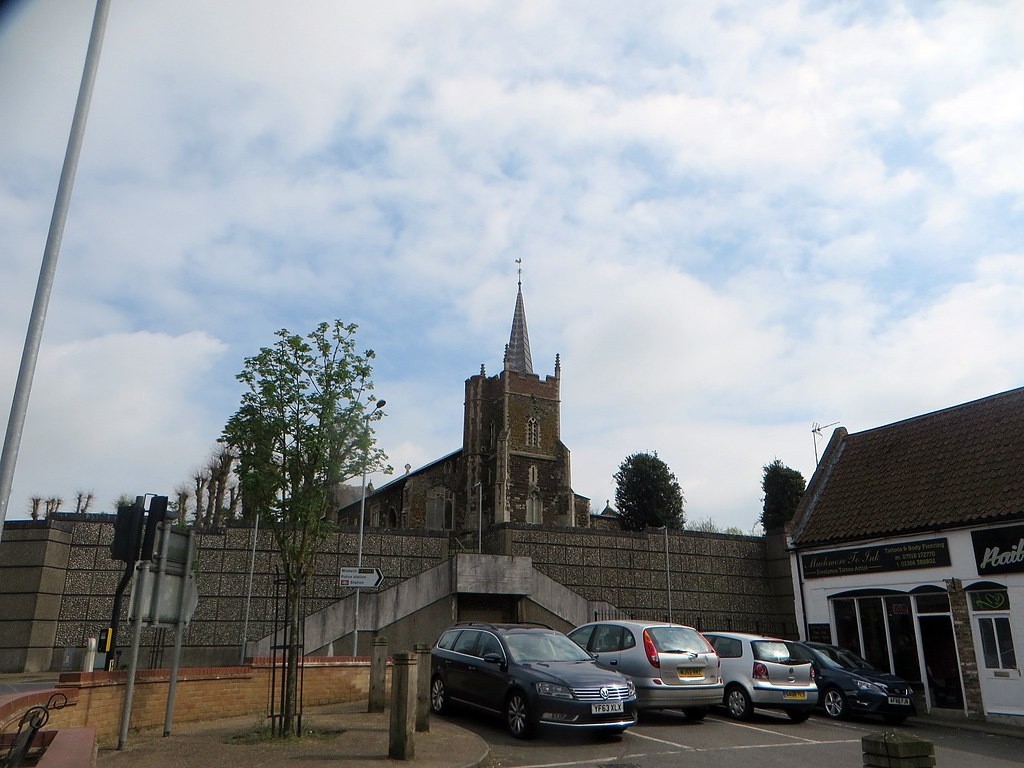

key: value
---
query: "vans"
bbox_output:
[785,639,914,724]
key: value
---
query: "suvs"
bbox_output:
[566,620,719,721]
[430,621,638,740]
[699,632,818,723]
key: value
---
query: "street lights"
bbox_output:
[350,400,387,656]
